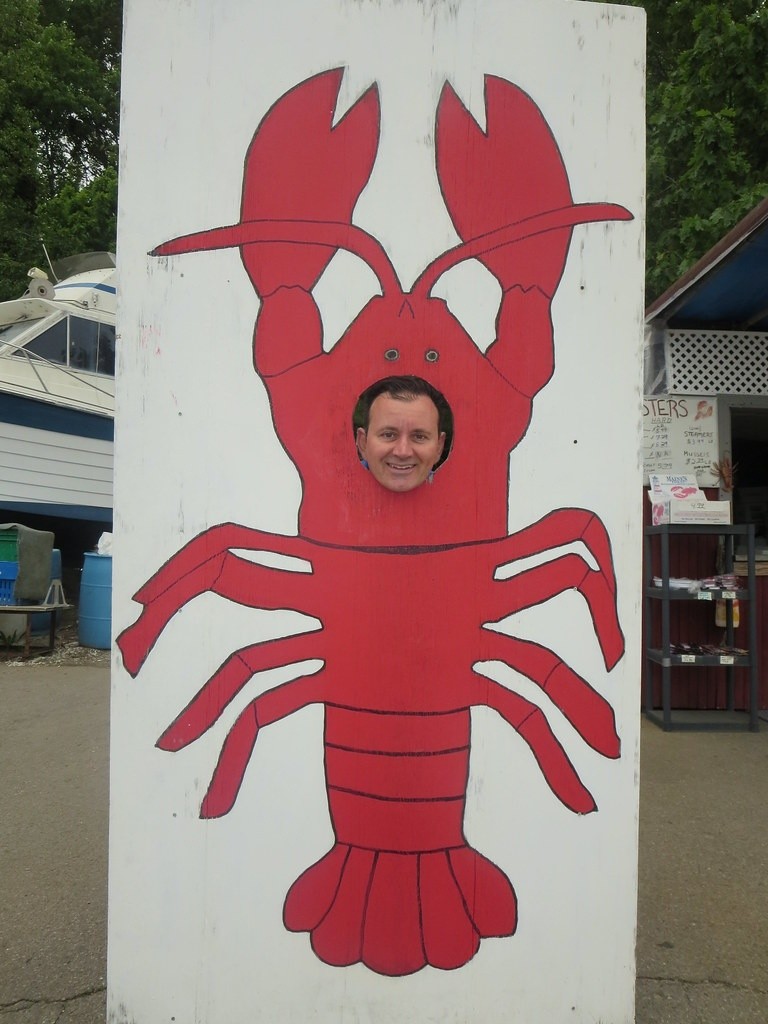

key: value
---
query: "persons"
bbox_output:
[357,377,445,492]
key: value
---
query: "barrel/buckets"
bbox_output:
[78,552,112,651]
[30,548,62,630]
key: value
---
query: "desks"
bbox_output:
[0,603,74,661]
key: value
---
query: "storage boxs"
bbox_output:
[0,524,40,607]
[653,499,732,525]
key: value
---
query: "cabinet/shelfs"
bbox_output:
[645,524,761,734]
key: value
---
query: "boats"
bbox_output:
[0,236,115,557]
